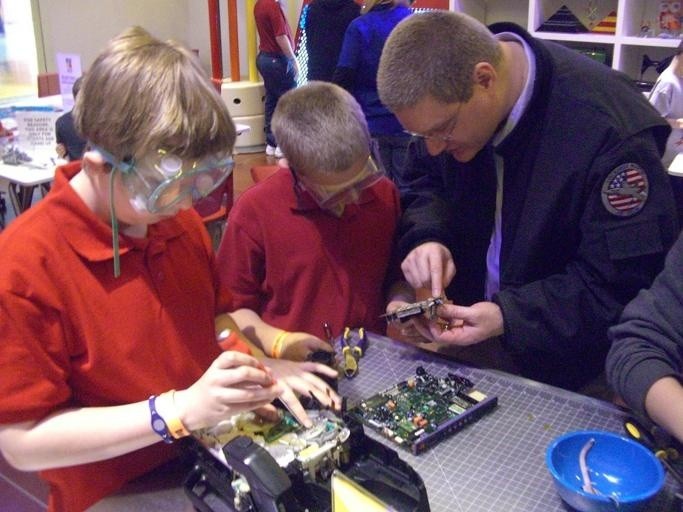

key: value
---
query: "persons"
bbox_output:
[252,1,301,158]
[305,0,361,83]
[375,8,682,392]
[328,0,415,179]
[216,80,402,365]
[0,26,345,512]
[602,227,682,446]
[649,37,683,175]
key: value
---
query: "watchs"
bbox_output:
[146,394,173,445]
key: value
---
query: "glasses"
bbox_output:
[101,144,235,219]
[403,75,469,142]
[287,137,386,209]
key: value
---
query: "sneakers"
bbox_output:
[266,144,275,155]
[275,147,285,158]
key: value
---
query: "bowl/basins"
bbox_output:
[544,429,665,512]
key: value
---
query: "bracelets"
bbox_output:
[160,389,189,440]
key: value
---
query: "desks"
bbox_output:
[84,329,644,512]
[0,141,71,216]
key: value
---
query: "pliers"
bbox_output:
[340,326,367,380]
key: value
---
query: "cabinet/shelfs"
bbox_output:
[527,0,683,177]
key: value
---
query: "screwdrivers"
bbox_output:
[322,321,336,354]
[216,327,304,426]
[622,417,683,485]
